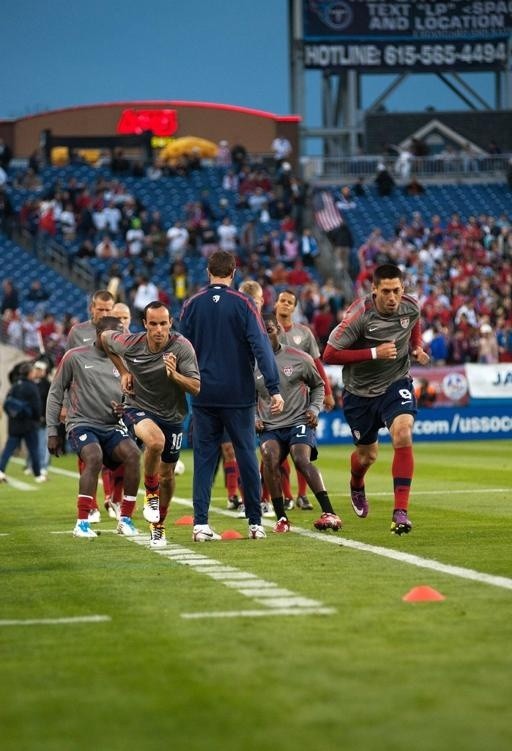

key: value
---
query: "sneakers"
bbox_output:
[193,525,221,541]
[109,499,121,520]
[390,511,412,536]
[351,479,368,518]
[143,493,160,523]
[117,516,137,536]
[88,508,100,523]
[149,523,165,547]
[104,499,116,518]
[225,498,343,538]
[73,519,97,537]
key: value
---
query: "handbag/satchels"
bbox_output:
[4,397,32,419]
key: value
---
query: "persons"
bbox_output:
[322,263,432,537]
[46,291,200,550]
[44,316,144,540]
[237,285,336,513]
[99,299,202,550]
[352,102,500,197]
[1,280,86,486]
[9,167,165,264]
[176,249,286,544]
[249,310,344,536]
[165,134,351,283]
[356,213,511,363]
